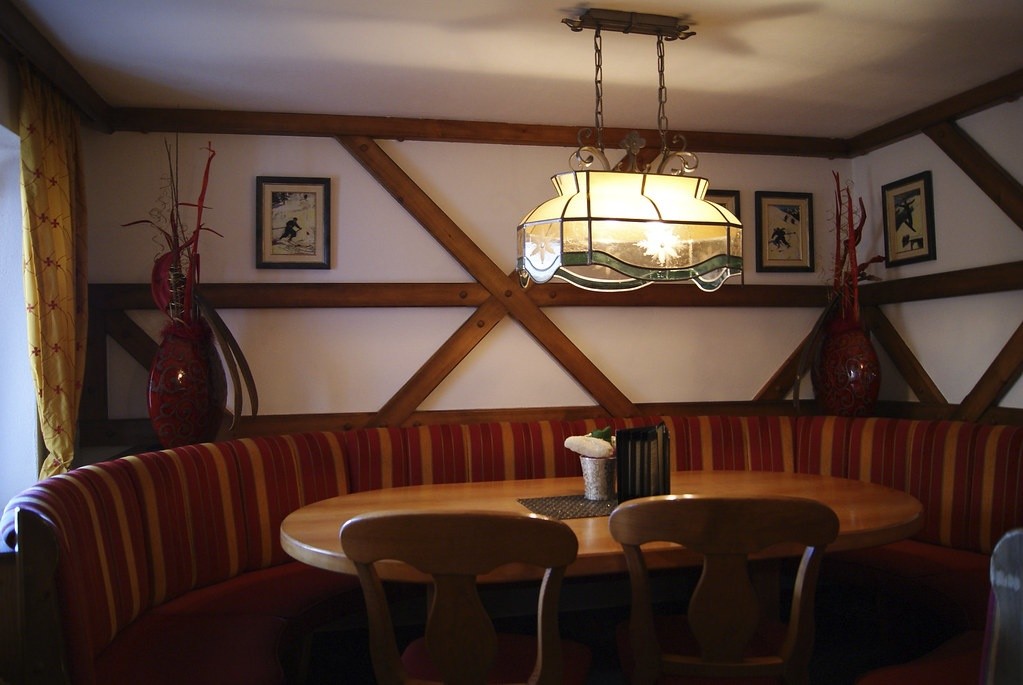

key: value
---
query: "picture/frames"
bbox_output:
[255,176,331,270]
[881,170,937,268]
[706,188,741,221]
[754,190,815,273]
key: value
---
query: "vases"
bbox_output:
[811,321,881,416]
[146,335,227,449]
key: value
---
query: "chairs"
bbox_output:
[612,492,840,685]
[339,508,596,685]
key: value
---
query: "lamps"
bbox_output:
[515,8,743,293]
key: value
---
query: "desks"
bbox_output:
[280,471,926,623]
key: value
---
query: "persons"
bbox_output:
[279,218,300,240]
[772,228,789,248]
[898,199,916,226]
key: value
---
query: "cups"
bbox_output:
[579,456,617,501]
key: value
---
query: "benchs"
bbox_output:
[1,415,1023,685]
